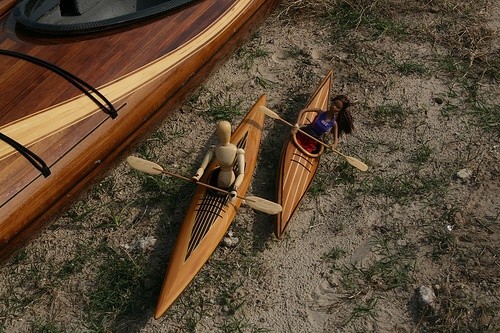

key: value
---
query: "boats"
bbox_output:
[155,93,266,319]
[278,71,334,239]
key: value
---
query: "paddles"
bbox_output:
[127,156,282,214]
[258,106,368,171]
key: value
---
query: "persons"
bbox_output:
[193,120,245,201]
[291,96,357,152]
